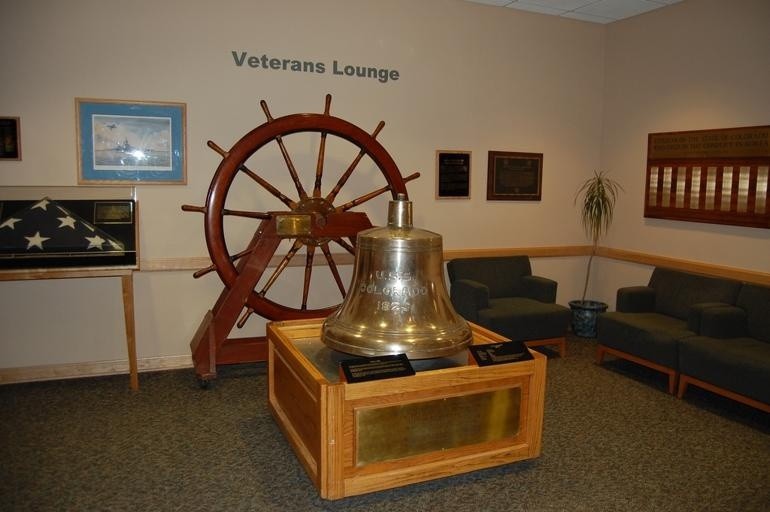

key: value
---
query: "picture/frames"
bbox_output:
[436,148,543,203]
[0,115,22,163]
[72,94,189,187]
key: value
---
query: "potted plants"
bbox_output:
[569,168,626,339]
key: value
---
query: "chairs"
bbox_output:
[594,265,770,421]
[445,255,569,362]
